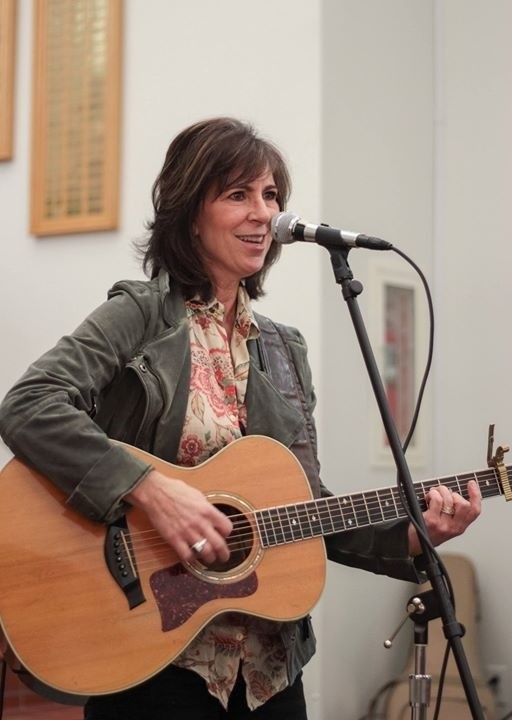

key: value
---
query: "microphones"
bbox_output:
[271,213,393,250]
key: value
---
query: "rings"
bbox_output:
[442,505,455,515]
[192,537,207,554]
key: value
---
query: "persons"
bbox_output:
[0,118,482,720]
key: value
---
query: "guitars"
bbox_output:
[0,428,511,709]
